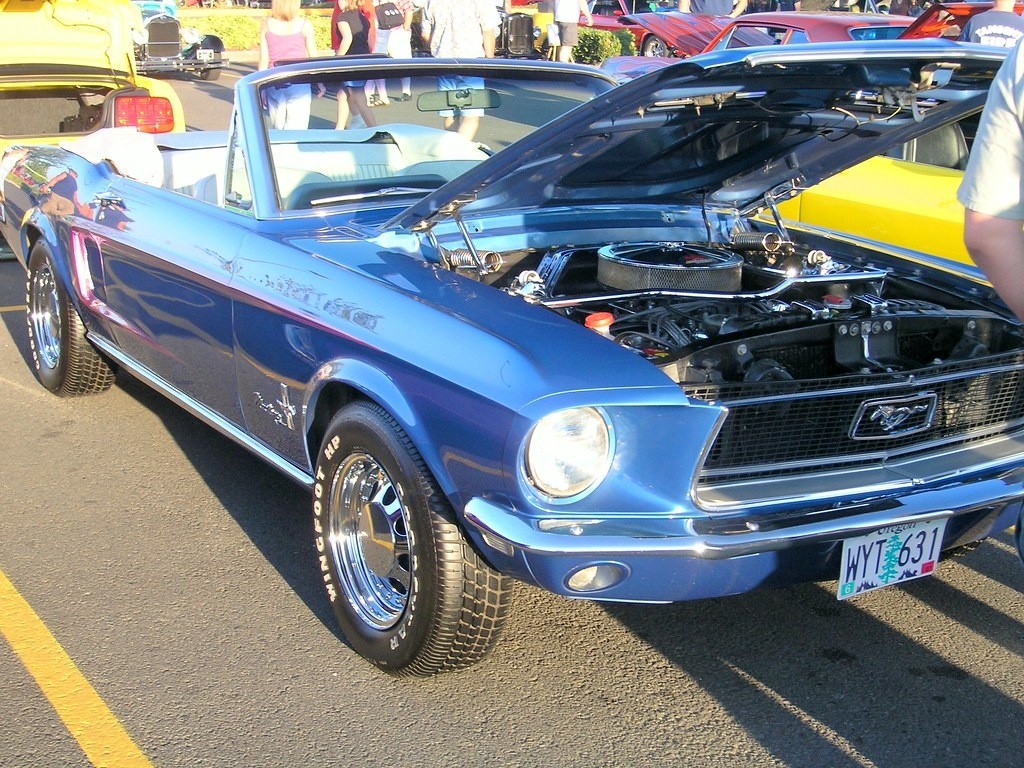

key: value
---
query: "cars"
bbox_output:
[698,0,998,44]
[175,0,389,52]
[1,36,1023,679]
[119,0,223,80]
[758,98,1000,282]
[579,1,777,63]
[1,0,184,257]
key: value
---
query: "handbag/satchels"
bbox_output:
[376,3,404,29]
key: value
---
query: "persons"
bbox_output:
[775,0,803,12]
[907,0,924,17]
[257,0,327,131]
[330,0,413,107]
[957,35,1024,327]
[889,0,910,16]
[334,0,377,131]
[421,0,503,141]
[955,0,1024,49]
[554,0,593,63]
[677,0,748,18]
[40,168,82,216]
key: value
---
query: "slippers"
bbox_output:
[371,100,390,106]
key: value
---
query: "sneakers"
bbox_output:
[347,115,367,131]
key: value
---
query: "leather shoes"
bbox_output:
[398,93,412,102]
